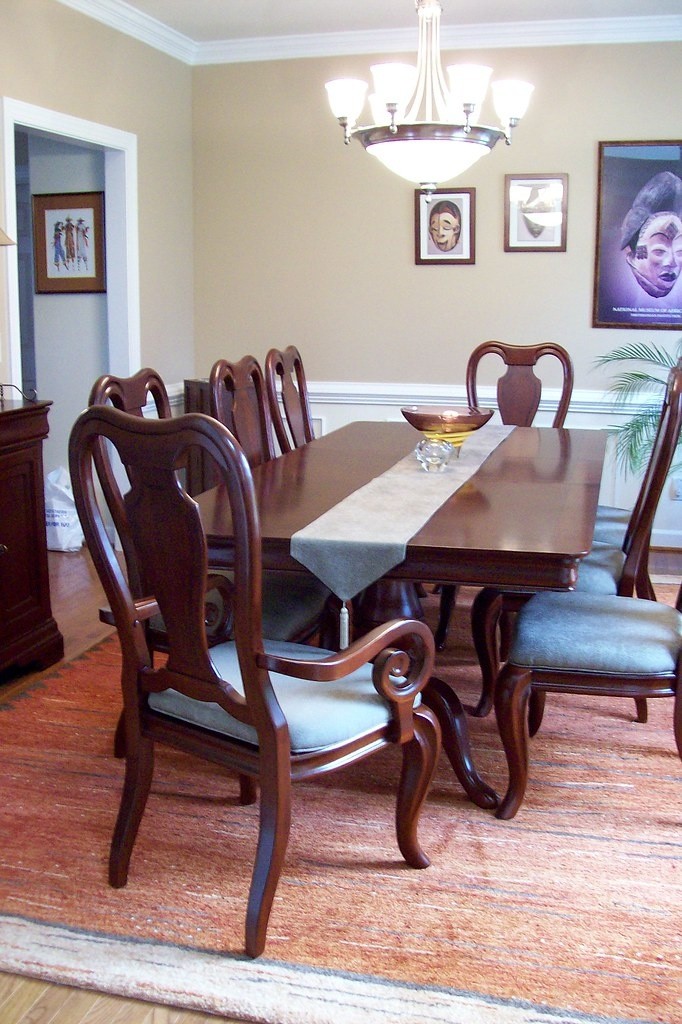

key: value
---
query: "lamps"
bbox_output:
[322,0,535,202]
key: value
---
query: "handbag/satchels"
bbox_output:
[44,465,89,553]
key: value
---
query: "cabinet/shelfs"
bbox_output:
[0,397,64,671]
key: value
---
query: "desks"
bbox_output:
[192,422,608,811]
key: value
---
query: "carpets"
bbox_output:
[0,575,682,1024]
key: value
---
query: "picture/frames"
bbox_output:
[505,173,569,252]
[415,188,475,265]
[31,191,107,294]
[590,139,682,331]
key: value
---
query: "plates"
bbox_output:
[400,405,495,432]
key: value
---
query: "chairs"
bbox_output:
[68,340,682,960]
